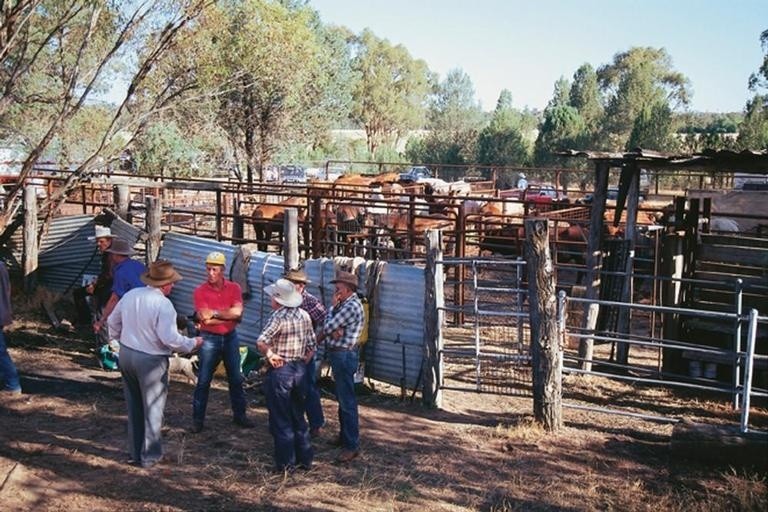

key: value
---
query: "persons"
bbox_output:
[191,251,255,433]
[86,224,118,308]
[316,270,366,462]
[286,269,329,435]
[106,259,203,469]
[92,240,149,334]
[0,261,23,396]
[256,278,318,472]
[517,173,528,201]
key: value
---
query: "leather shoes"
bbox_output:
[188,421,200,433]
[243,419,257,427]
[337,447,362,461]
[251,400,266,409]
[310,427,324,438]
[327,437,340,445]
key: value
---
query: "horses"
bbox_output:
[252,197,305,254]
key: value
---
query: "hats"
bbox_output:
[263,277,303,307]
[140,259,183,287]
[104,236,136,255]
[87,228,117,240]
[328,270,359,288]
[206,251,226,265]
[284,267,309,284]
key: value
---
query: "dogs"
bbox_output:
[168,356,198,385]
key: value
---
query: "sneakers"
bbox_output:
[0,387,21,396]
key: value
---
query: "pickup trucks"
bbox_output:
[501,183,569,210]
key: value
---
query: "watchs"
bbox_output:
[213,309,219,317]
